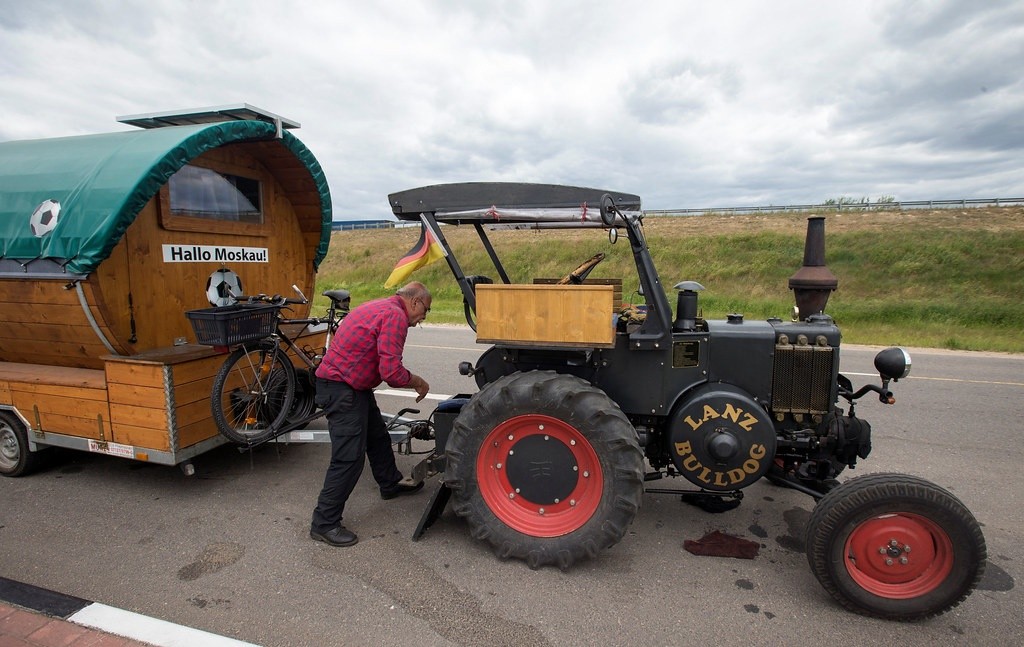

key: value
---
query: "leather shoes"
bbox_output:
[310,521,357,545]
[381,479,425,500]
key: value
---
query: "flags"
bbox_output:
[383,213,448,289]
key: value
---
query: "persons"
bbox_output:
[310,281,433,547]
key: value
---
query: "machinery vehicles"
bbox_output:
[0,100,988,624]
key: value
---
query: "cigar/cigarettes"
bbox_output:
[418,321,424,331]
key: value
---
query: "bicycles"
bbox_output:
[185,284,353,444]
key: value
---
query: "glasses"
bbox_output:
[412,297,431,315]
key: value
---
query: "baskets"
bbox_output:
[185,303,281,345]
